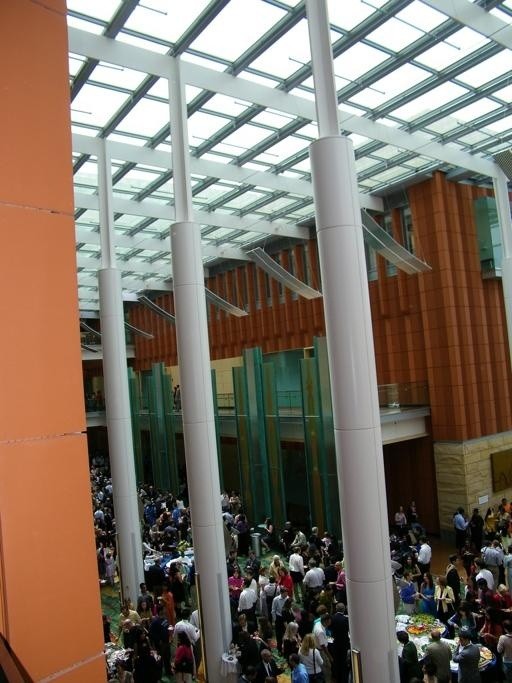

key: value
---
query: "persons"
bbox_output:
[103,498,512,683]
[173,385,181,412]
[89,457,250,621]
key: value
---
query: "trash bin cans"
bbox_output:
[251,533,261,557]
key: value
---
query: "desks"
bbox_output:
[89,462,511,682]
